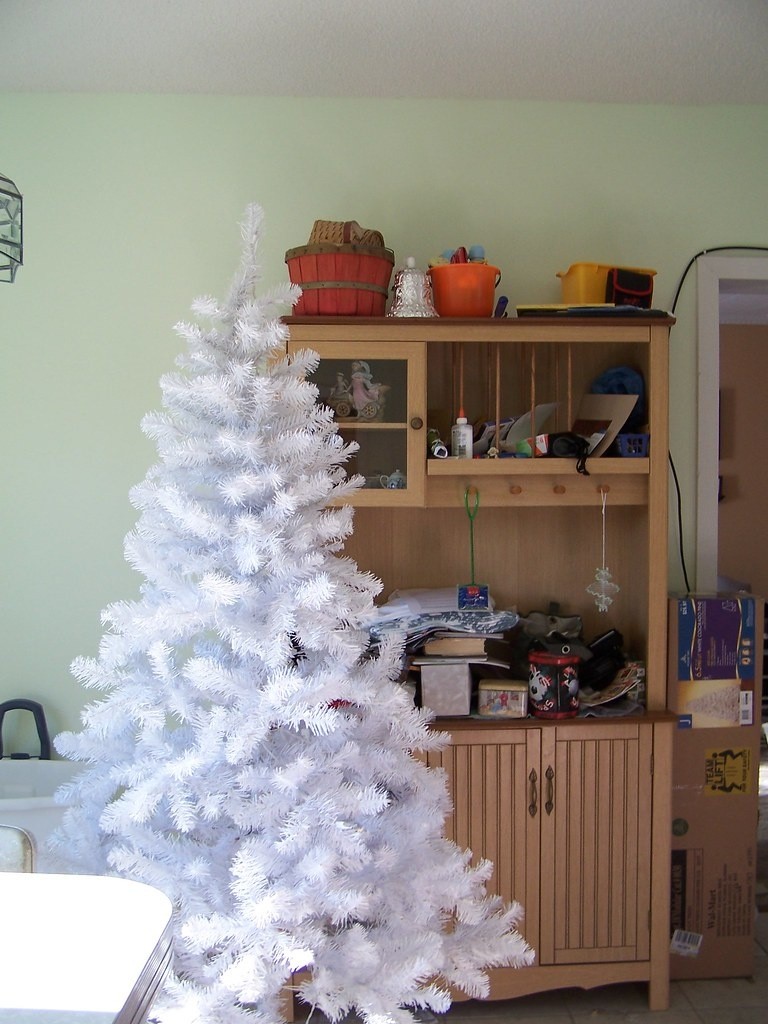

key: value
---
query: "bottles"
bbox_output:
[451,407,473,458]
[388,244,486,318]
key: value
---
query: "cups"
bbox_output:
[380,469,406,489]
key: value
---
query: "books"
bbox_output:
[370,583,510,669]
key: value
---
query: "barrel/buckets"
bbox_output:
[427,266,501,318]
[284,244,395,318]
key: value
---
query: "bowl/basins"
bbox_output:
[356,473,379,488]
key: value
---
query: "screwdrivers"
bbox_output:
[495,296,508,318]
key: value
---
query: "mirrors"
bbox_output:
[696,256,768,725]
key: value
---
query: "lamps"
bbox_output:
[0,172,23,282]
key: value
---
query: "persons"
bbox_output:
[333,360,382,418]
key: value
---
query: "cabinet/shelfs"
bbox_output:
[259,315,673,1023]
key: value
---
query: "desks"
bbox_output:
[0,872,176,1024]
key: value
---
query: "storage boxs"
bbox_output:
[558,263,655,307]
[665,591,762,980]
